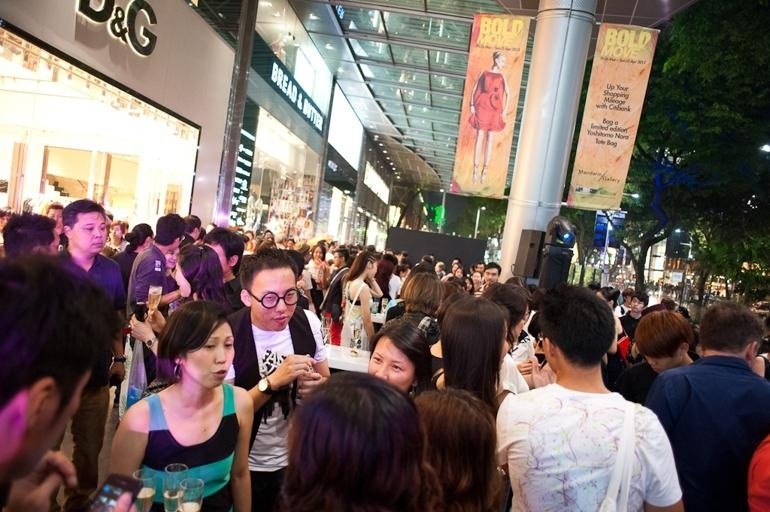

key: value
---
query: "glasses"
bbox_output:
[538,332,555,346]
[356,340,361,348]
[244,288,300,309]
[180,234,185,241]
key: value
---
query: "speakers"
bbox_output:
[512,230,546,278]
[539,244,572,289]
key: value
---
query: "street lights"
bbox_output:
[675,227,695,259]
[601,191,641,273]
[473,205,488,238]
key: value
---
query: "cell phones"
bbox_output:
[81,473,143,512]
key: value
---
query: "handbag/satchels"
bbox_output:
[318,262,330,290]
[340,283,367,350]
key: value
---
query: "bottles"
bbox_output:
[295,364,314,406]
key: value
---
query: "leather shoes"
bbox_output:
[63,496,91,512]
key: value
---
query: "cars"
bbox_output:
[754,300,770,310]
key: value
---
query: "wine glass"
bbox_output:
[133,467,157,510]
[163,462,188,511]
[176,478,204,510]
[146,285,163,319]
[320,312,365,357]
[381,298,388,312]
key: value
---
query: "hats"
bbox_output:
[295,243,310,255]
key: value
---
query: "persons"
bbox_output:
[285,241,769,512]
[1,199,332,512]
[468,52,510,183]
